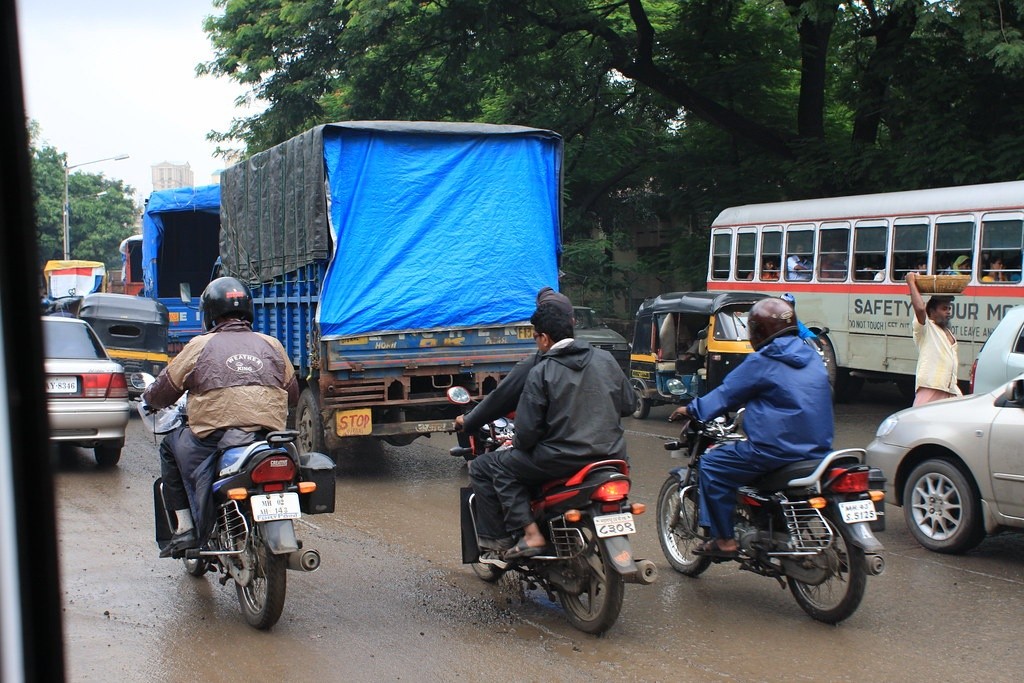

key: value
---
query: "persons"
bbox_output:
[780,293,826,345]
[984,255,1021,282]
[143,277,300,558]
[788,243,814,280]
[763,258,774,278]
[456,287,575,568]
[672,297,834,557]
[917,258,927,275]
[937,254,971,275]
[822,247,846,278]
[905,272,963,406]
[493,304,638,559]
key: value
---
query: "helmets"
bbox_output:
[780,293,795,310]
[198,277,254,331]
[747,297,799,352]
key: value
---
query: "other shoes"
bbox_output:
[159,529,198,558]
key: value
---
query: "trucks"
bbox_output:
[43,260,107,316]
[118,233,144,296]
[209,119,566,475]
[138,183,228,357]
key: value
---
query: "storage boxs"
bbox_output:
[866,468,886,531]
[299,454,336,515]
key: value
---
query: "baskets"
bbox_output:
[905,273,971,293]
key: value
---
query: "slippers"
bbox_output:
[502,538,543,559]
[692,538,737,559]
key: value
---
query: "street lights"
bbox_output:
[63,150,131,259]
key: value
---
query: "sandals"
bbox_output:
[478,550,512,569]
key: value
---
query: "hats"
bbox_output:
[537,286,575,320]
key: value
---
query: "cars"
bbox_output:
[41,315,129,468]
[967,306,1024,399]
[863,372,1024,556]
[570,304,631,371]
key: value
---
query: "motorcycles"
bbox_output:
[129,371,338,631]
[626,291,774,420]
[657,378,889,625]
[76,293,169,403]
[446,386,659,634]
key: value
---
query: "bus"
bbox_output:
[705,177,1024,406]
